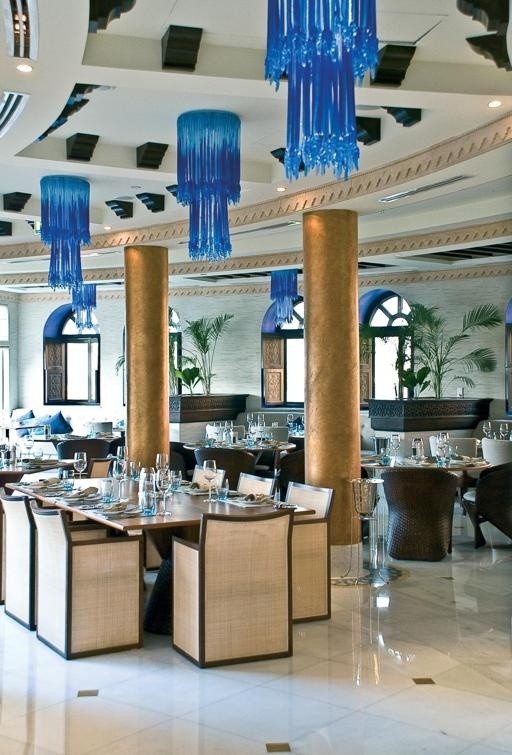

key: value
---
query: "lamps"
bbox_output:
[270,270,299,328]
[264,0,382,183]
[40,176,97,330]
[175,109,243,265]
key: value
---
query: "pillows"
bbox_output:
[14,410,75,438]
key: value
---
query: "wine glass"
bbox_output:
[212,410,305,447]
[0,434,58,470]
[14,446,297,521]
[390,420,509,468]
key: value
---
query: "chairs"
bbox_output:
[191,465,225,487]
[462,462,512,551]
[236,473,277,497]
[3,420,512,484]
[0,487,96,632]
[379,467,459,562]
[173,508,295,670]
[290,481,336,626]
[29,500,145,661]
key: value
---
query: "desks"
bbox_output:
[5,477,316,634]
[340,477,389,587]
